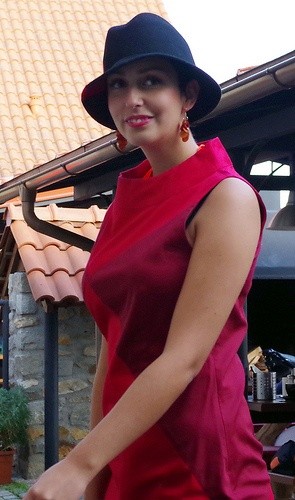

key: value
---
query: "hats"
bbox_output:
[81,13,222,130]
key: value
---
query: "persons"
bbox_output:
[25,12,275,500]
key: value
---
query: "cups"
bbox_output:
[285,383,295,396]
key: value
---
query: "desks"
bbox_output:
[243,394,295,448]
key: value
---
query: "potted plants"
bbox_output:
[0,382,35,486]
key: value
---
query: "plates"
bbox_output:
[279,395,295,401]
